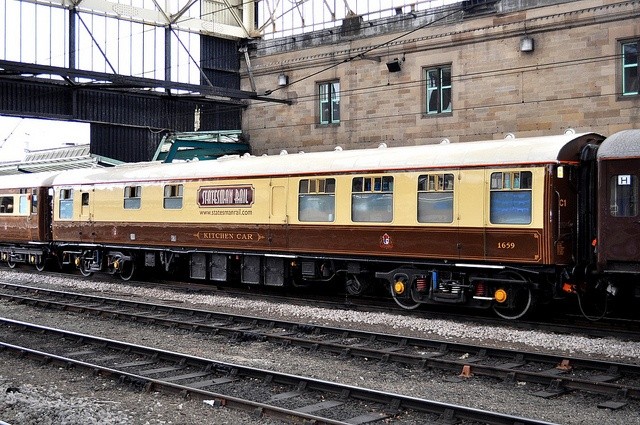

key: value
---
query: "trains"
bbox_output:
[0,129,639,331]
